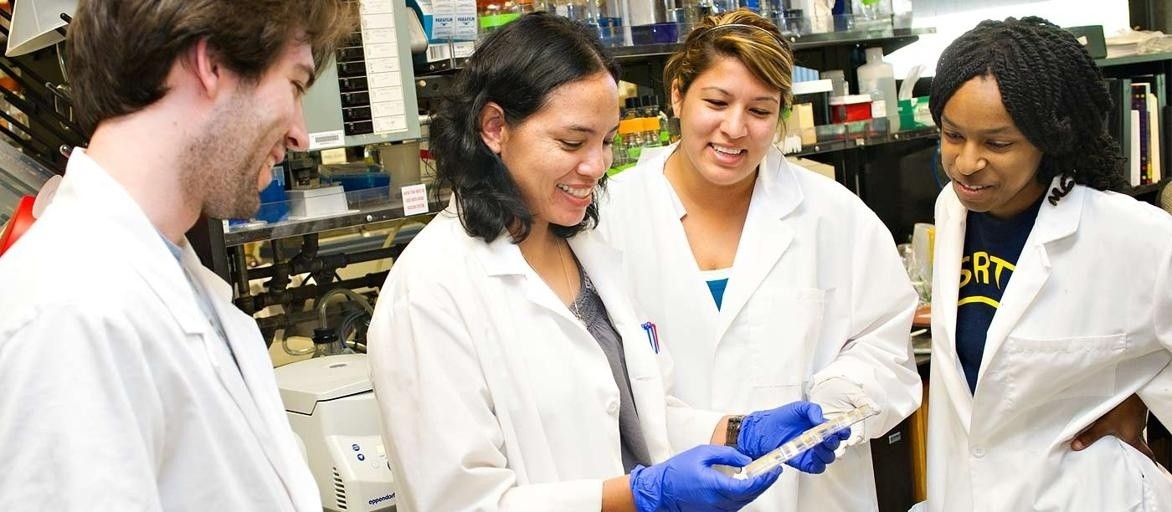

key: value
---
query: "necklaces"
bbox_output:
[520,237,589,332]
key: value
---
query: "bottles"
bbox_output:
[614,95,672,167]
[480,0,898,46]
[827,45,901,145]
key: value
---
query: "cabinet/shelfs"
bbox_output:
[410,23,944,238]
[222,128,436,354]
[1074,24,1171,199]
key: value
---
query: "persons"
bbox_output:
[1,0,367,512]
[367,14,851,512]
[590,7,923,512]
[924,13,1172,511]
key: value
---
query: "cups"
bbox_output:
[898,243,929,287]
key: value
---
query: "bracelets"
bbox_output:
[726,414,746,450]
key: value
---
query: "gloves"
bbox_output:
[736,401,851,475]
[629,444,783,512]
